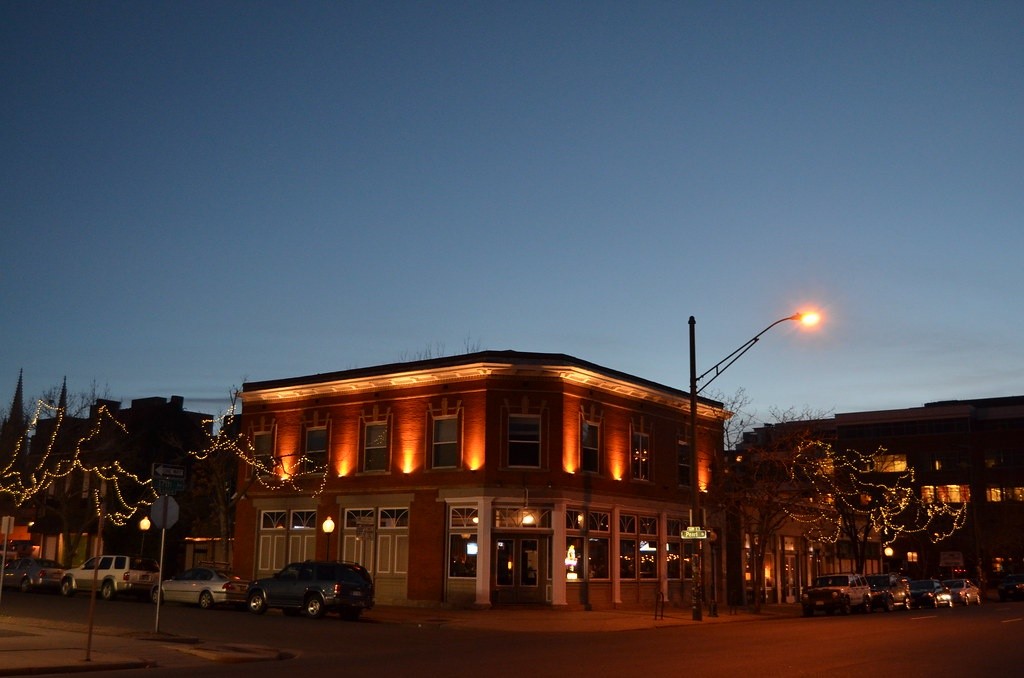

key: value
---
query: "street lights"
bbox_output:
[884,545,893,574]
[688,309,823,622]
[322,516,335,561]
[138,516,150,569]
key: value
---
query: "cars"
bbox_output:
[0,558,68,593]
[940,579,981,606]
[998,574,1024,601]
[908,580,952,610]
[150,567,251,610]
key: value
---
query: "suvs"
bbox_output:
[865,572,912,613]
[58,554,162,601]
[800,573,871,616]
[246,559,375,618]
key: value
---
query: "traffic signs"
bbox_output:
[681,530,707,539]
